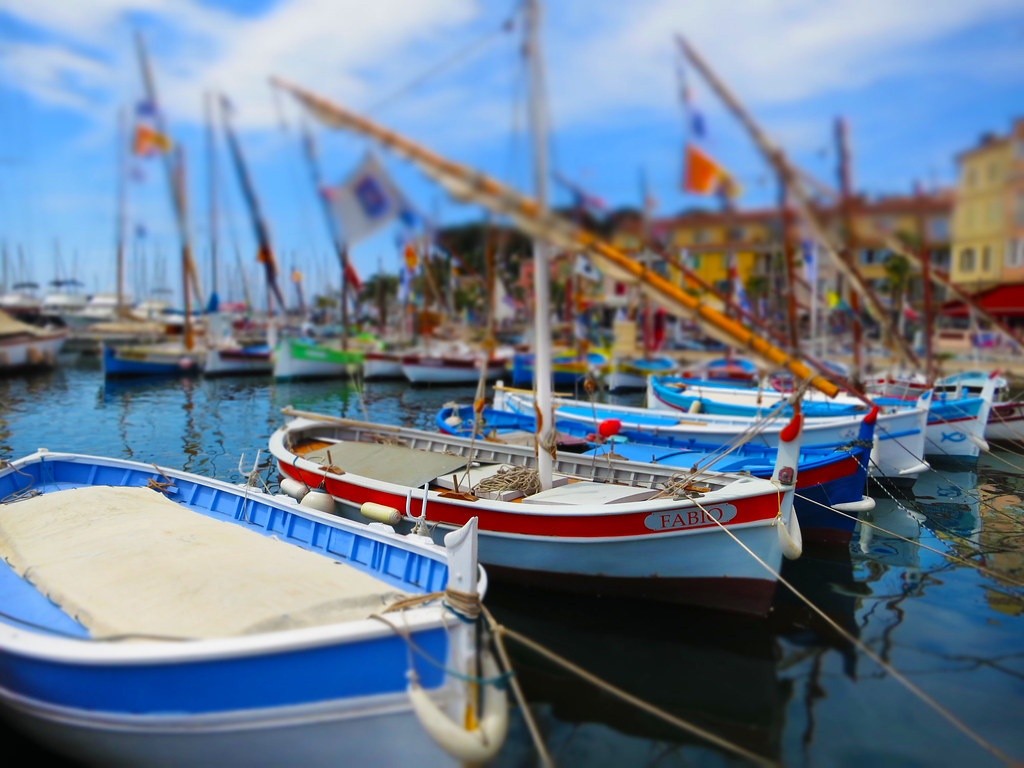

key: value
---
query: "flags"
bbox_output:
[131,102,172,161]
[323,157,405,249]
[681,82,743,200]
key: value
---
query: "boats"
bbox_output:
[0,447,486,768]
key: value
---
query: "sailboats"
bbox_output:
[1,0,1023,551]
[265,0,808,623]
